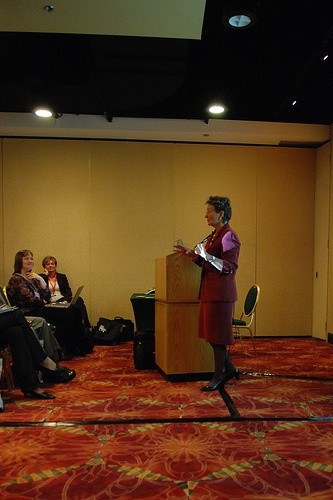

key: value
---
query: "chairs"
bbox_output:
[0,286,17,393]
[229,284,260,356]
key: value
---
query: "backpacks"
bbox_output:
[133,329,158,370]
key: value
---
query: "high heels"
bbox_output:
[24,390,56,399]
[39,362,69,375]
[224,368,240,382]
[201,375,226,392]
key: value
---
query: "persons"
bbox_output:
[37,256,91,329]
[173,195,241,392]
[0,287,76,388]
[7,249,56,333]
[0,305,70,400]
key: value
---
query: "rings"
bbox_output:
[182,248,184,250]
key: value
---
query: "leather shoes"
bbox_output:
[41,369,76,383]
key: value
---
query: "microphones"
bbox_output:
[201,235,211,243]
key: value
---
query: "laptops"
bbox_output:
[45,285,84,308]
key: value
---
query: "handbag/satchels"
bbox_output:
[112,317,134,342]
[92,317,123,345]
